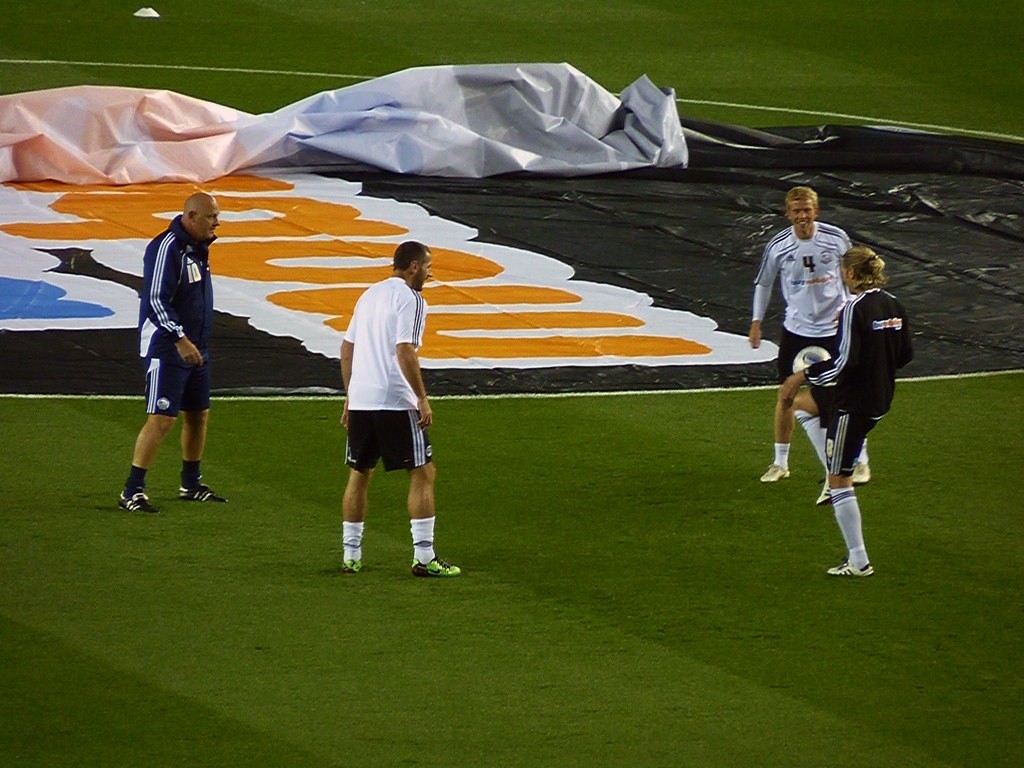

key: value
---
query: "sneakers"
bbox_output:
[118,486,160,514]
[760,464,790,482]
[411,556,462,576]
[827,556,874,577]
[851,463,872,487]
[342,560,362,573]
[178,483,229,505]
[816,475,833,506]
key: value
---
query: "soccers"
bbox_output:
[792,346,832,374]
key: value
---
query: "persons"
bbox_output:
[749,186,871,485]
[117,193,229,513]
[340,241,461,577]
[778,246,913,577]
